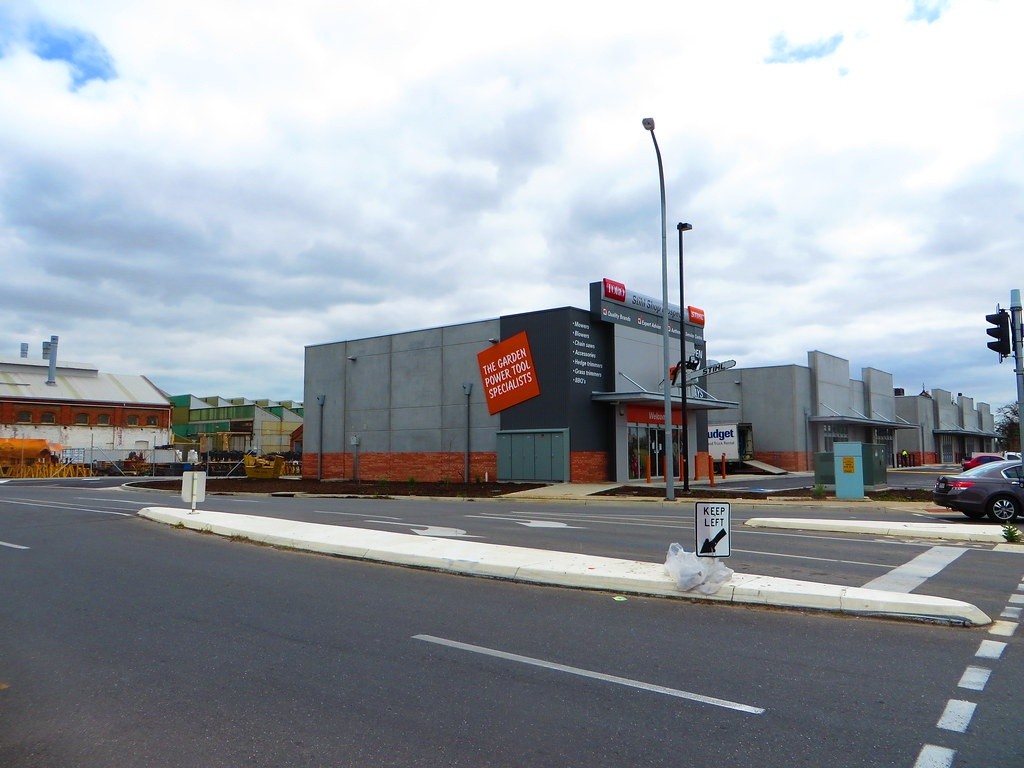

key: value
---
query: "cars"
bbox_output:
[961,454,1007,472]
[931,460,1024,524]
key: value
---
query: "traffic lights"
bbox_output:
[986,312,1010,355]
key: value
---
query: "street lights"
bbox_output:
[642,117,676,500]
[677,221,692,490]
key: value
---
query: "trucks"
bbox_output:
[971,450,1022,460]
